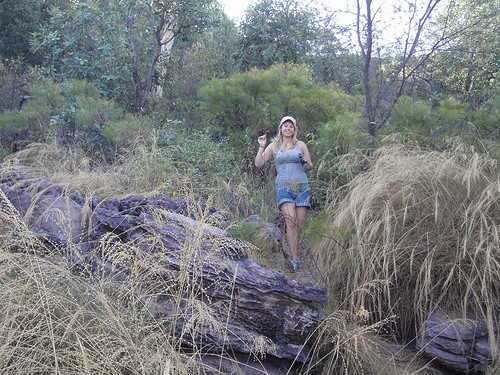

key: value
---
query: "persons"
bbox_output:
[255,115,313,273]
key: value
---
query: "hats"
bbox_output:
[280,116,296,126]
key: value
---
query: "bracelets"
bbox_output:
[259,144,265,148]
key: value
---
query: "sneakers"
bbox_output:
[292,260,299,270]
